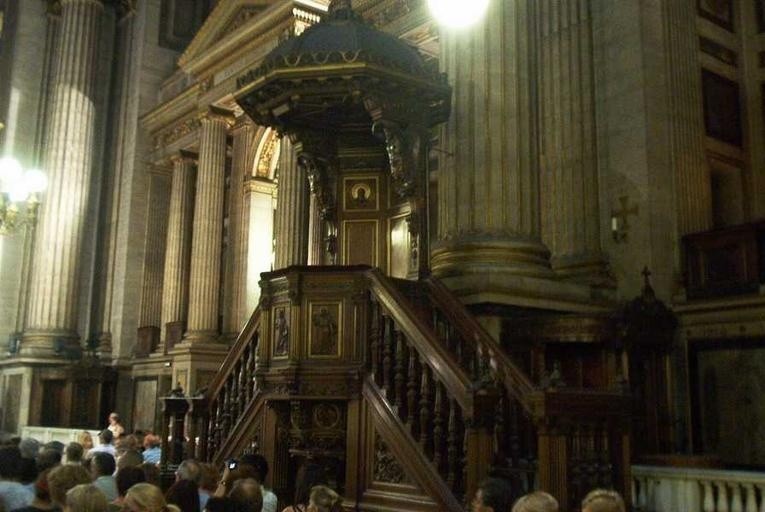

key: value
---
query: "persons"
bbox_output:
[1,414,343,511]
[582,487,625,510]
[470,478,518,511]
[509,488,560,511]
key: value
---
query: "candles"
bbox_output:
[612,216,617,231]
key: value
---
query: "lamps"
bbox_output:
[0,155,50,234]
